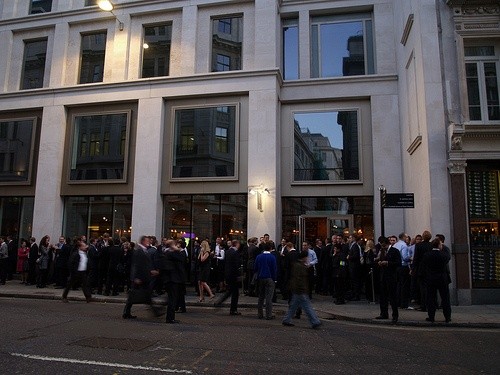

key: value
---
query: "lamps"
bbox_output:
[97,0,123,31]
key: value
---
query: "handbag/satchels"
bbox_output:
[23,256,29,271]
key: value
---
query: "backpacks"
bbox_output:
[335,255,347,273]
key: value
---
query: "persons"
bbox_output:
[253,242,278,320]
[374,236,402,323]
[0,229,453,328]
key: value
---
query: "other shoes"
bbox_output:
[122,315,137,319]
[376,316,389,319]
[229,312,242,316]
[166,320,180,324]
[282,322,295,327]
[266,316,275,320]
[392,317,398,321]
